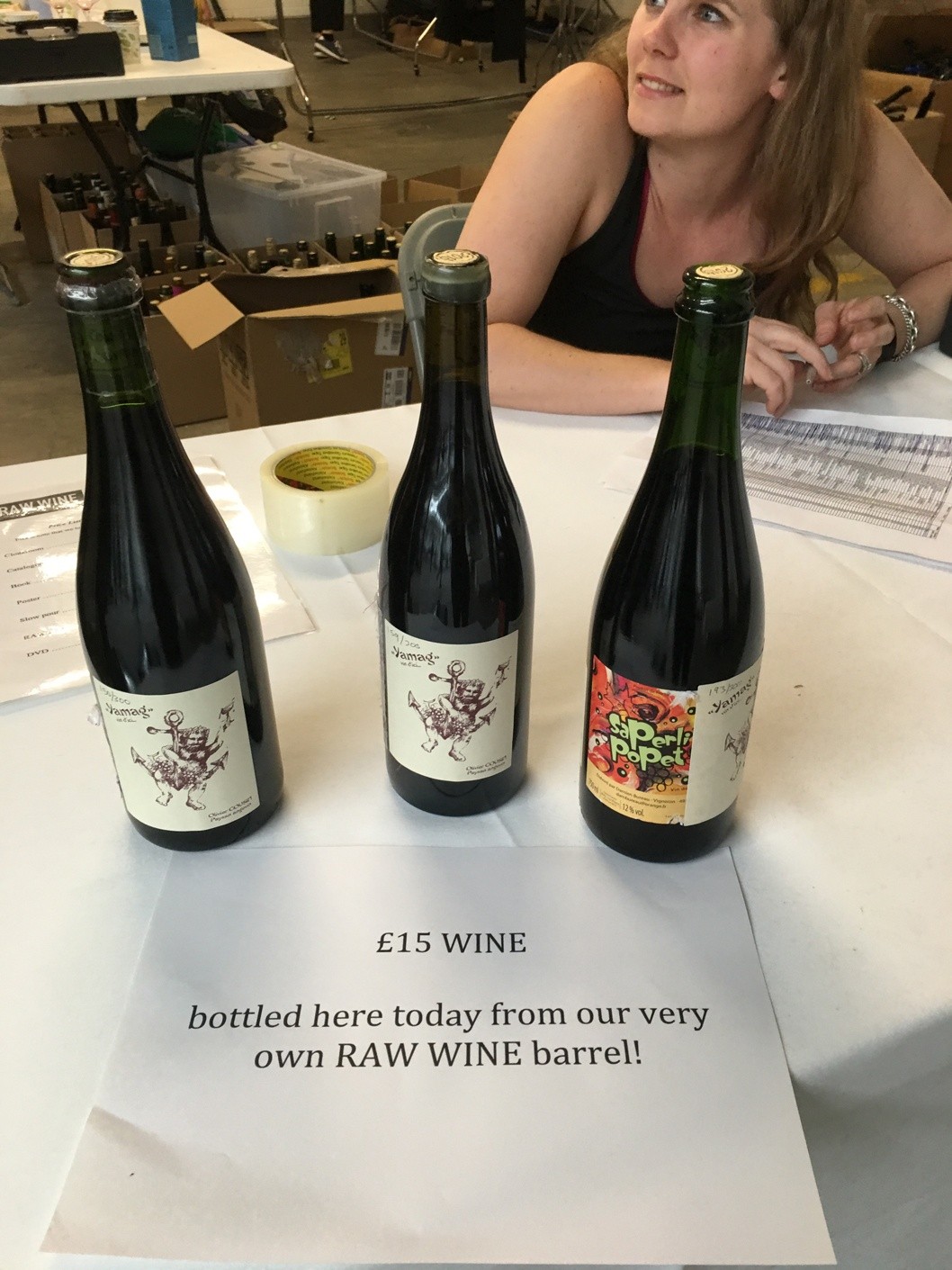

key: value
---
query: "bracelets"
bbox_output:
[875,295,919,365]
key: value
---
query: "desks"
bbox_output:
[1,21,295,253]
[0,398,952,1264]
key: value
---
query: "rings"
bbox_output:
[851,351,873,376]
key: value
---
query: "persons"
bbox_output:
[309,0,349,63]
[454,0,952,418]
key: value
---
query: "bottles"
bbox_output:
[43,167,412,310]
[52,247,283,854]
[580,262,768,867]
[380,248,528,818]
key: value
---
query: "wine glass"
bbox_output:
[42,0,70,18]
[75,0,96,20]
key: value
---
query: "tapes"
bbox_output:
[261,442,386,553]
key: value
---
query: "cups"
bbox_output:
[102,9,141,64]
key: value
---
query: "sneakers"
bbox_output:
[314,36,349,63]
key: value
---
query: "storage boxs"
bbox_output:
[858,7,952,199]
[1,119,492,423]
[388,14,491,70]
[141,0,199,61]
